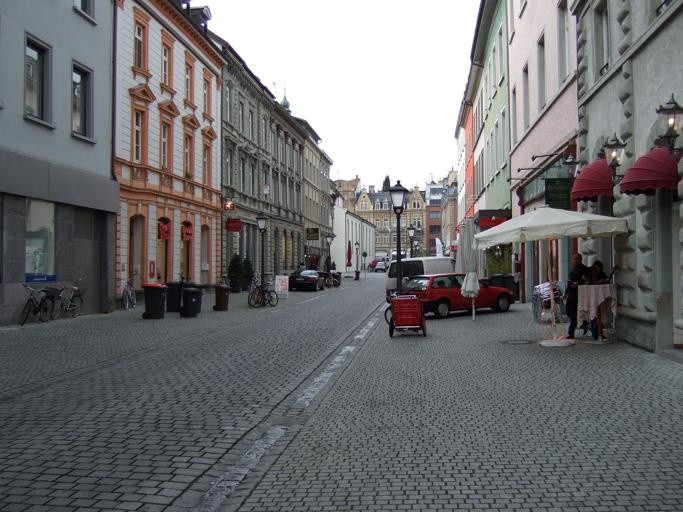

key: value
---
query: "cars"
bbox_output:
[375,258,389,274]
[401,274,518,318]
[287,268,322,291]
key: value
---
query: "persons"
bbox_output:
[346,241,352,276]
[563,253,591,338]
[588,258,608,283]
[437,281,445,288]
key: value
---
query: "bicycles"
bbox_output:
[326,269,340,287]
[246,278,278,309]
[46,278,85,321]
[18,282,55,324]
[119,277,139,310]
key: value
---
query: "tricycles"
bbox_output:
[384,289,428,336]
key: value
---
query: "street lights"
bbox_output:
[257,209,268,285]
[407,223,415,258]
[389,178,409,292]
[326,233,334,269]
[415,245,420,257]
[414,238,420,257]
[354,241,359,271]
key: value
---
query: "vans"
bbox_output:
[384,256,456,301]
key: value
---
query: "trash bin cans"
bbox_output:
[317,272,342,287]
[141,281,232,319]
[355,271,360,280]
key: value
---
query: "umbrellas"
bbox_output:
[476,206,627,341]
[456,216,480,321]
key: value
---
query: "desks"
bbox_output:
[576,284,619,346]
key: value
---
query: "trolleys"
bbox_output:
[533,278,567,326]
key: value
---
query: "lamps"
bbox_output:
[504,91,682,185]
